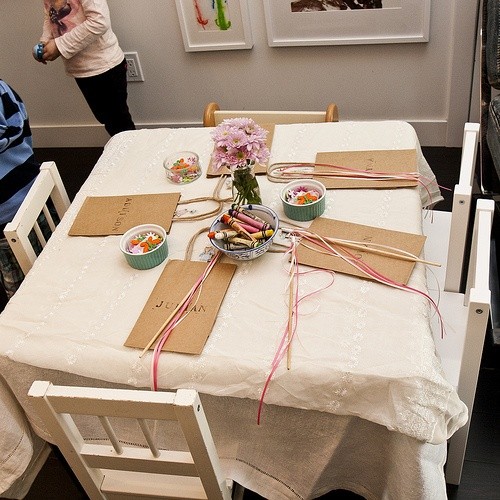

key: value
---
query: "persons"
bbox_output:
[0,78,54,300]
[33,0,136,137]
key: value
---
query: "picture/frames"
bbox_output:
[263,0,431,48]
[174,0,256,53]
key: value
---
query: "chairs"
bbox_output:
[425,121,483,289]
[4,158,74,277]
[201,101,339,128]
[24,373,243,500]
[418,192,497,489]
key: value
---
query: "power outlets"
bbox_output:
[124,51,145,82]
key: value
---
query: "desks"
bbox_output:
[0,118,471,500]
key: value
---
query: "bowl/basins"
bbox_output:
[163,150,202,184]
[209,204,279,261]
[119,224,168,269]
[279,179,327,221]
